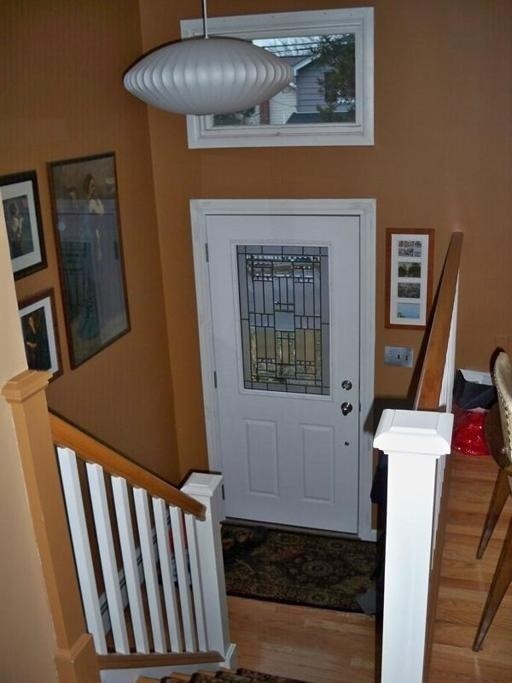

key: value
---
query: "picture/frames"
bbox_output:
[176,7,377,148]
[386,227,432,329]
[0,151,131,383]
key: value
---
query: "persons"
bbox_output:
[78,172,128,339]
[25,314,47,370]
[9,201,35,257]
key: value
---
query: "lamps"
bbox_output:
[122,0,295,117]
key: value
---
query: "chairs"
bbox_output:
[473,347,512,654]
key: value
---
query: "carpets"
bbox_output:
[157,516,382,616]
